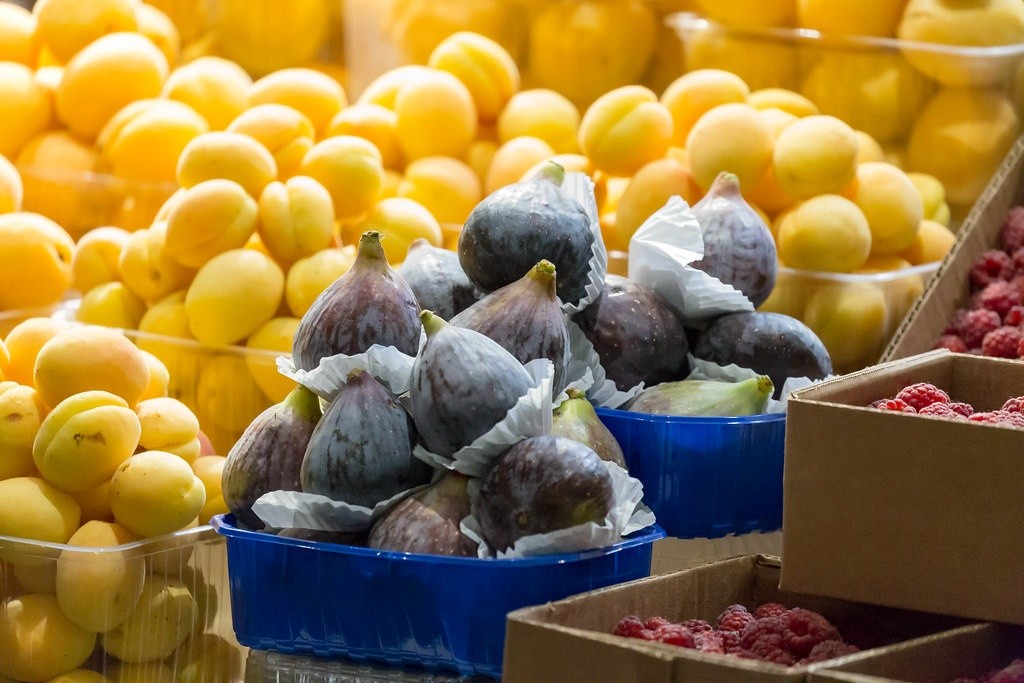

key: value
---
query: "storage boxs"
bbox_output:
[0,4,1024,683]
